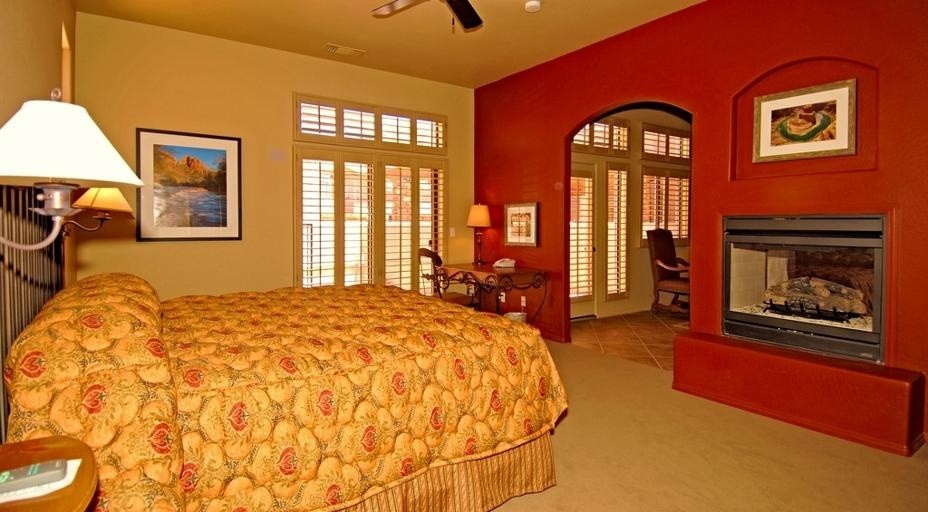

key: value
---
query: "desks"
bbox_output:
[441,264,548,326]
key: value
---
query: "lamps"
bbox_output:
[464,202,491,265]
[0,99,142,251]
[62,188,133,235]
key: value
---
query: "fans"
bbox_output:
[369,0,482,32]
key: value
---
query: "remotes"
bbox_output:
[0,457,68,494]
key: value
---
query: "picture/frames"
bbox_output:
[752,79,856,162]
[502,202,538,248]
[135,127,242,242]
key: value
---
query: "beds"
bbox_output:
[0,177,568,512]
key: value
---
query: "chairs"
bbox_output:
[646,228,690,317]
[417,248,480,310]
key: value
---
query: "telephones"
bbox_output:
[492,258,516,268]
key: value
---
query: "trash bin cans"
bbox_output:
[504,312,527,322]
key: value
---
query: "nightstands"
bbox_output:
[0,435,95,512]
